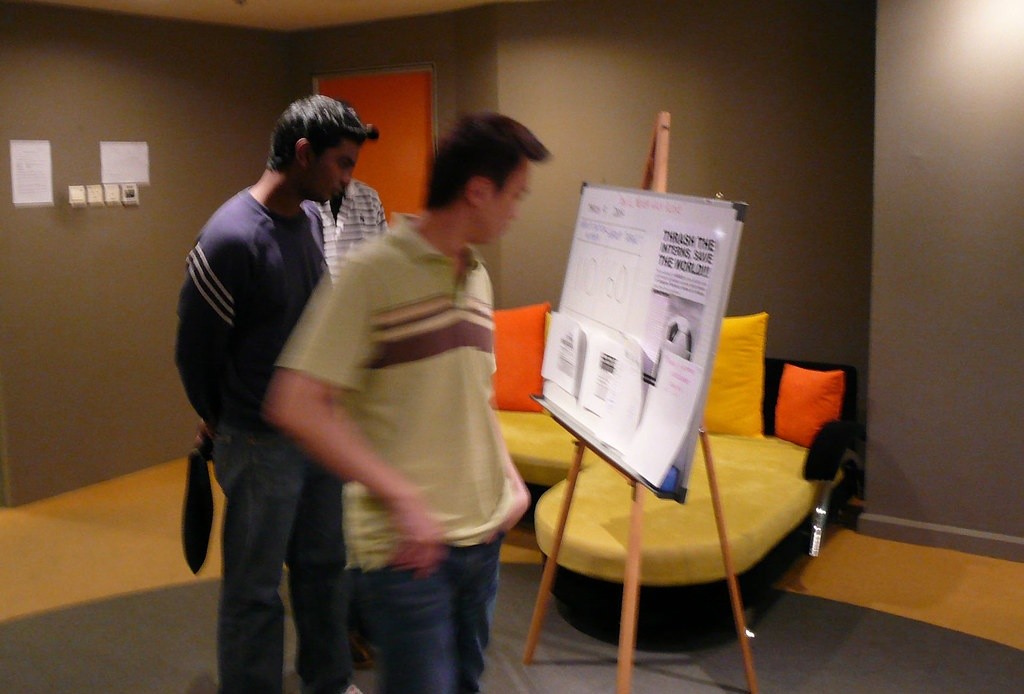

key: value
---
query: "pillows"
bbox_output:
[492,302,552,412]
[702,311,769,441]
[544,311,552,350]
[774,362,846,449]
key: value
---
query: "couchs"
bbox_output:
[489,356,867,653]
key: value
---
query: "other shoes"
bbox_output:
[299,678,362,694]
[349,605,389,642]
[348,626,378,668]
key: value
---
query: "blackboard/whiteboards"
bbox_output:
[527,179,749,505]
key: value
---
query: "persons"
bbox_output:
[263,112,552,694]
[178,95,389,694]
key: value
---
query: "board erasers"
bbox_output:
[645,463,680,494]
